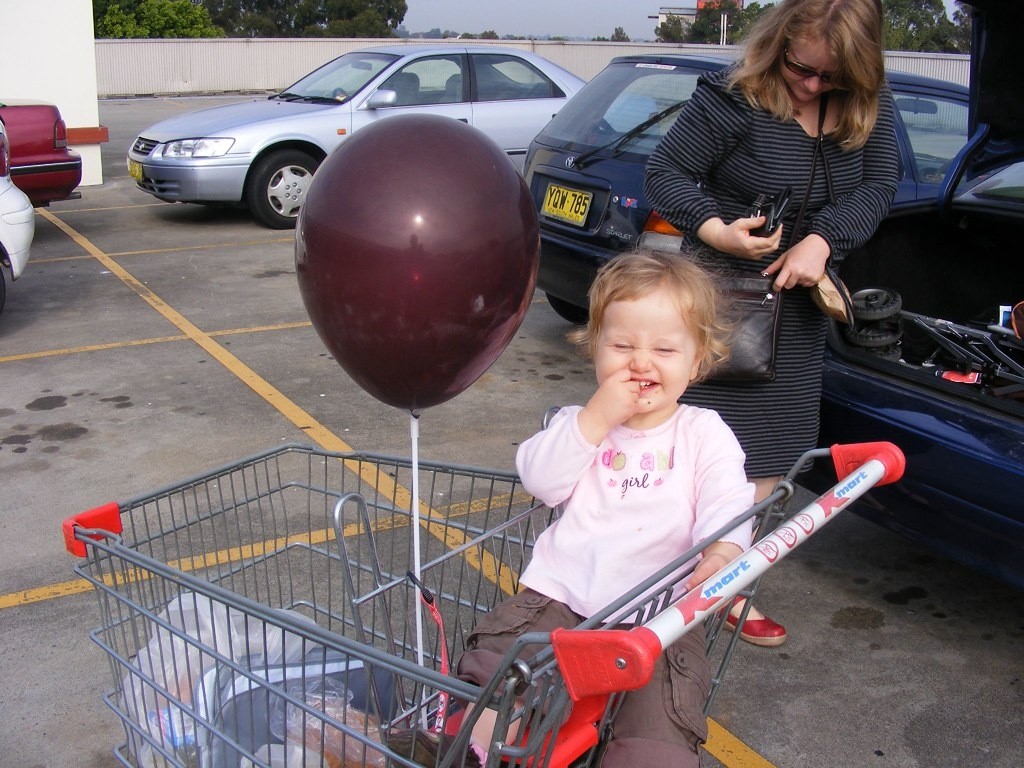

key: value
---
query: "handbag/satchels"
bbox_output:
[119,592,319,768]
[809,265,857,332]
[697,271,785,384]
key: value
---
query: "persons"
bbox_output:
[644,0,901,646]
[456,253,756,768]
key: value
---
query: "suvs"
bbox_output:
[519,52,974,327]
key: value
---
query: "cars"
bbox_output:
[1,120,36,315]
[0,102,83,207]
[126,42,659,231]
[789,0,1024,580]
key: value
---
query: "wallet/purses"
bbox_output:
[746,187,794,238]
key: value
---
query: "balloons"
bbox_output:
[295,115,540,418]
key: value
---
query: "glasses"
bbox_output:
[783,37,833,83]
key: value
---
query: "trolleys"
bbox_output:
[63,440,907,768]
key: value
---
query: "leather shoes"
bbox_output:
[716,595,787,646]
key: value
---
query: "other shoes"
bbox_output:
[387,728,482,768]
